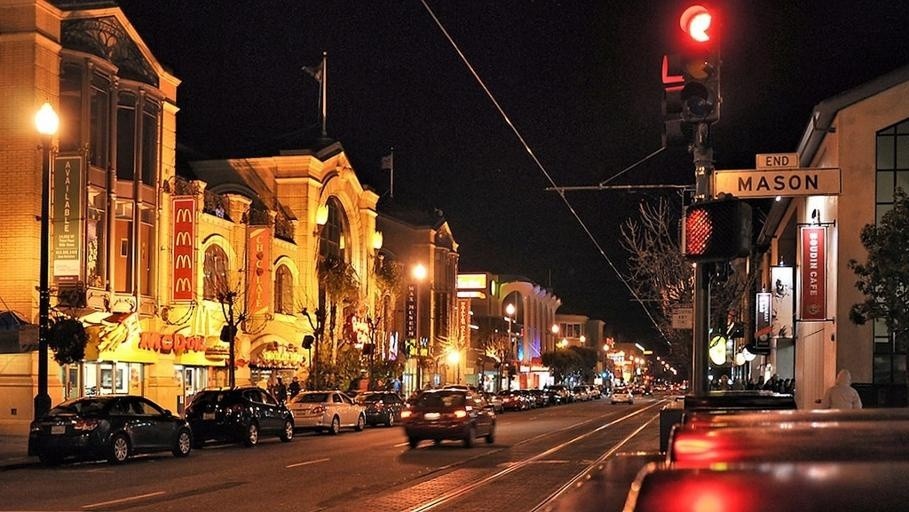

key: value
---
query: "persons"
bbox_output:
[821,367,865,411]
[710,371,794,394]
[287,376,302,400]
[275,378,287,400]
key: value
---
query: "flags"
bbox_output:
[379,155,392,171]
[301,62,323,84]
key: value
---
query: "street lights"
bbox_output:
[413,264,426,389]
[603,344,609,371]
[580,336,585,347]
[34,102,59,394]
[551,324,559,385]
[506,303,515,390]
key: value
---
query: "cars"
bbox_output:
[443,384,667,414]
[400,388,497,448]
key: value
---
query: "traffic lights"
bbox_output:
[681,199,715,260]
[662,3,720,120]
